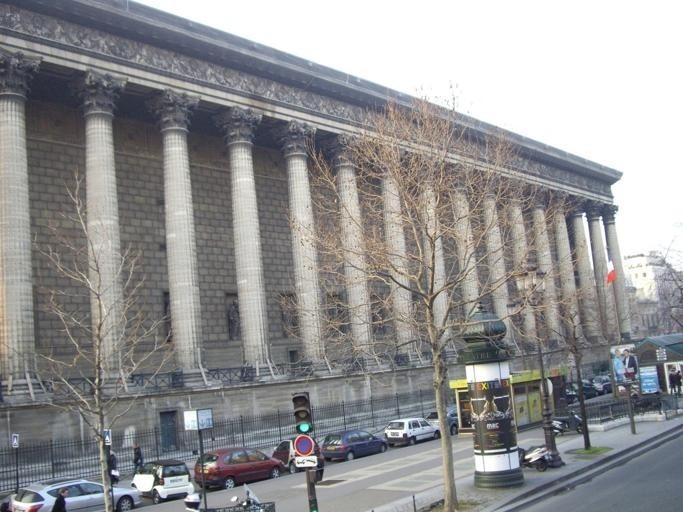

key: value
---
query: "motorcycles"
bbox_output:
[518,445,550,472]
[551,411,585,434]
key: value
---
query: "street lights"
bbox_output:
[513,250,558,451]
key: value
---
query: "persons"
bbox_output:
[669,367,682,395]
[133,442,145,477]
[614,348,637,386]
[52,488,69,512]
[109,446,118,484]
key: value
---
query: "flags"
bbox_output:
[606,260,617,283]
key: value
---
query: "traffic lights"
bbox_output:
[292,392,313,434]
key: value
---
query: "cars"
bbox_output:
[592,375,612,394]
[384,418,441,446]
[195,447,285,489]
[425,407,458,435]
[130,459,194,504]
[562,379,601,404]
[272,439,324,474]
[322,430,388,462]
[9,477,143,512]
